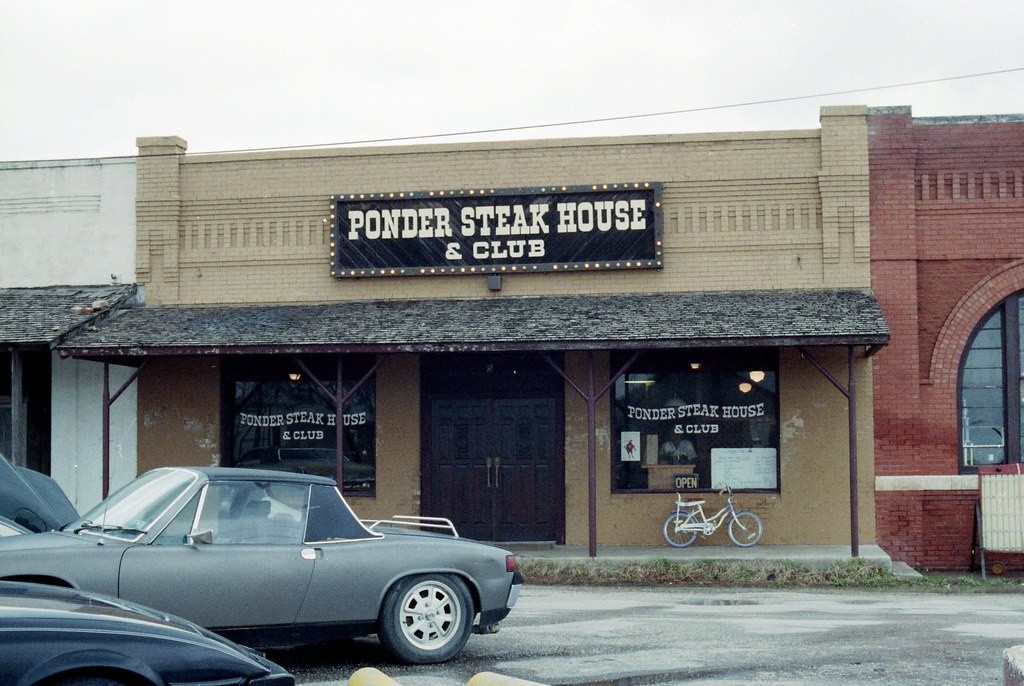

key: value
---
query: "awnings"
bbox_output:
[0,284,137,346]
[59,289,889,555]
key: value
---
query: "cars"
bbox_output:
[235,447,350,465]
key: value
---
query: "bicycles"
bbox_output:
[663,481,763,549]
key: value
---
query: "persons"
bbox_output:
[658,426,697,464]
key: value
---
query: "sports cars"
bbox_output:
[1,465,525,665]
[2,579,297,685]
